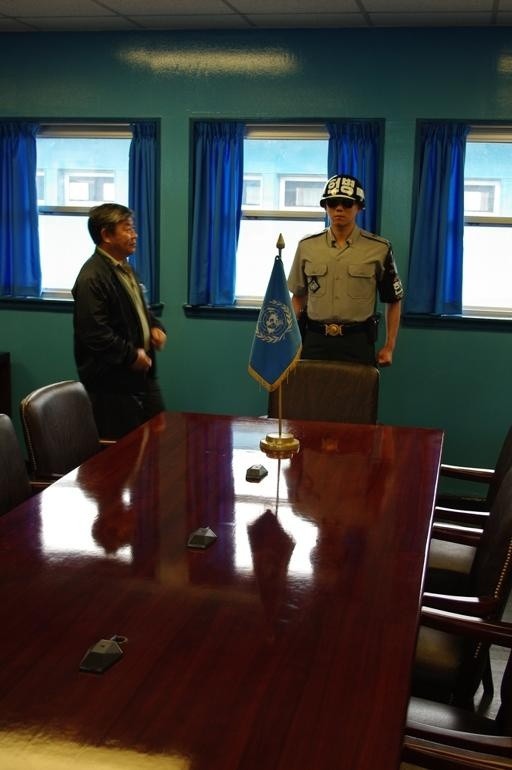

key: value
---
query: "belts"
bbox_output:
[308,321,366,337]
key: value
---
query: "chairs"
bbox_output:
[259,359,380,424]
[399,427,512,770]
[0,379,118,517]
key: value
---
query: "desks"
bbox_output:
[1,411,445,770]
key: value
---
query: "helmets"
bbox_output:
[319,174,367,210]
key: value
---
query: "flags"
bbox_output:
[248,253,302,389]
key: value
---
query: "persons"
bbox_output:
[281,418,400,595]
[74,203,168,445]
[75,409,165,552]
[285,173,407,369]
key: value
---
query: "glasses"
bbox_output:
[325,198,353,209]
